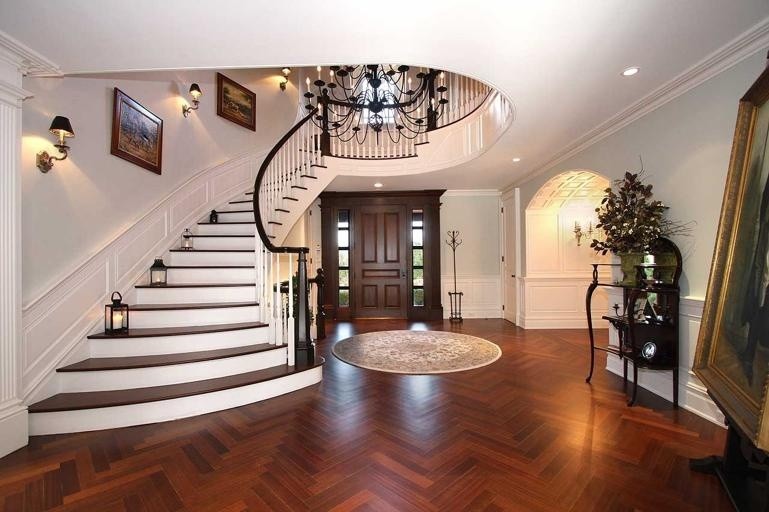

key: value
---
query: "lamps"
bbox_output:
[33,114,75,173]
[302,65,449,146]
[277,65,294,94]
[182,79,203,118]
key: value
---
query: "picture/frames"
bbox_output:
[688,64,769,454]
[107,86,165,177]
[215,69,259,133]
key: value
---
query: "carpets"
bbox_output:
[330,327,503,376]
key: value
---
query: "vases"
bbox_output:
[619,249,651,288]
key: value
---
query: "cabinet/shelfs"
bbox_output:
[583,239,682,412]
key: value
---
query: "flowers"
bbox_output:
[569,154,700,263]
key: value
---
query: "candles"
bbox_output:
[156,279,161,283]
[112,316,123,329]
